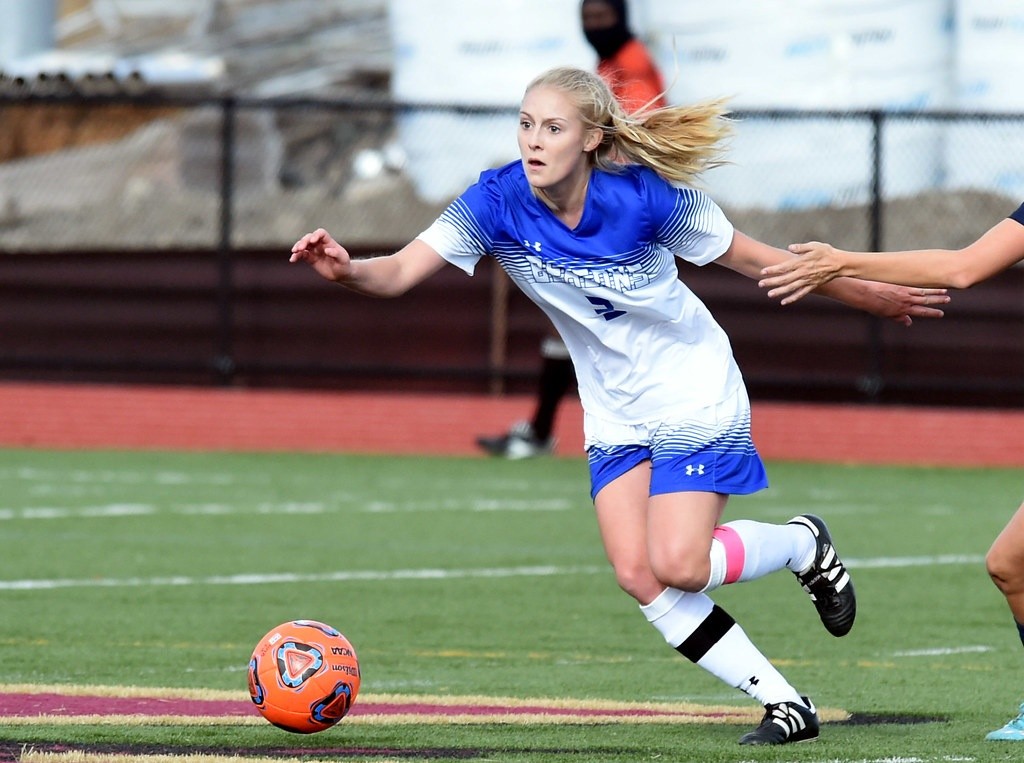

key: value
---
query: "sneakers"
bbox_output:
[476,432,548,458]
[784,513,856,637]
[986,704,1024,740]
[738,696,819,747]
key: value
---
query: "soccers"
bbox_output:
[248,619,362,735]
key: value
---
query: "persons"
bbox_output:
[471,0,672,461]
[289,66,949,752]
[757,196,1023,743]
